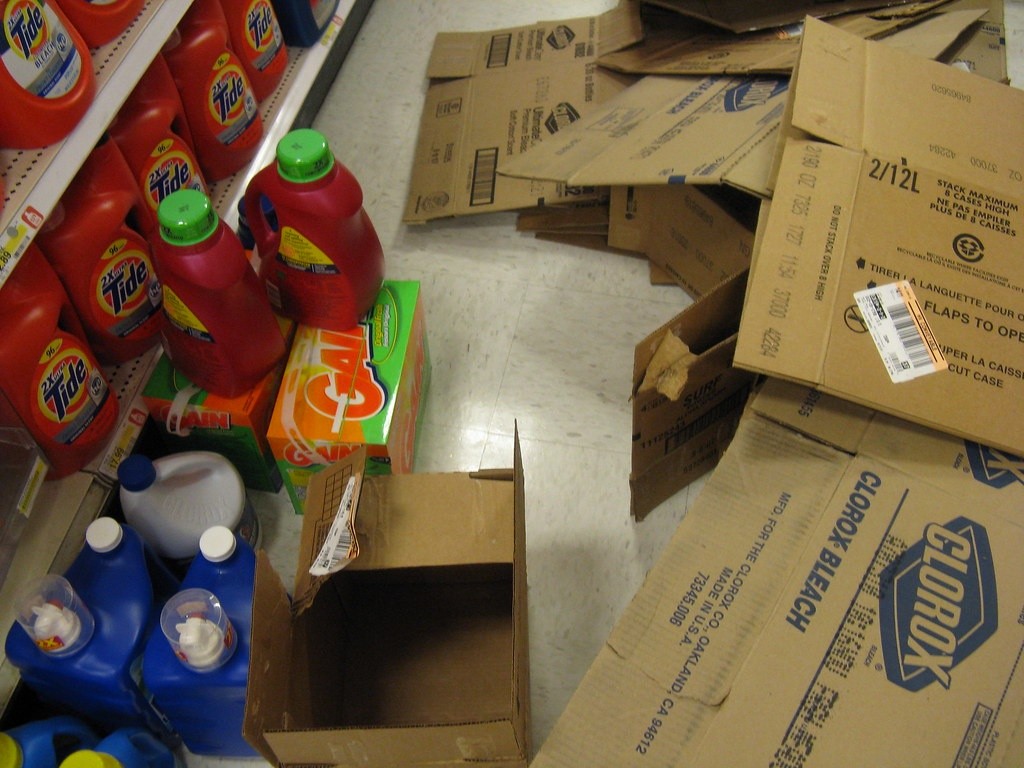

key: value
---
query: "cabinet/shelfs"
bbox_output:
[0,0,380,768]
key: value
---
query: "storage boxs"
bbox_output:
[242,418,535,767]
[141,312,297,495]
[265,280,432,515]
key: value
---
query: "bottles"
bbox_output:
[0,0,344,478]
[149,190,287,400]
[0,449,254,768]
[244,128,386,332]
